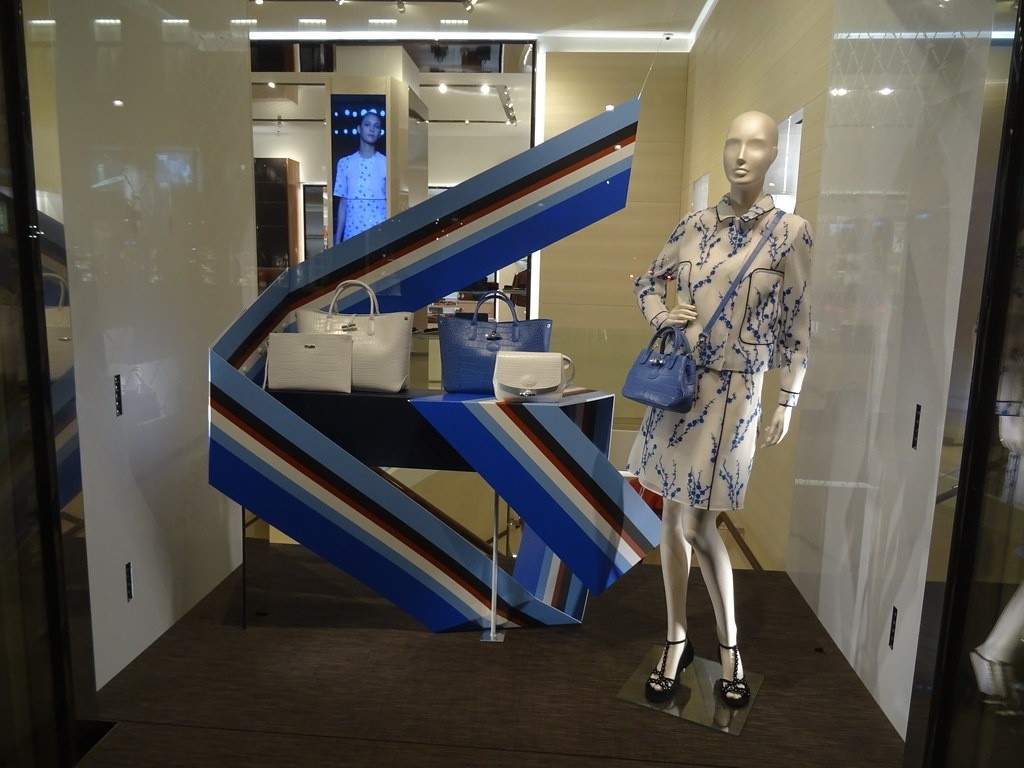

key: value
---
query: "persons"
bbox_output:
[627,111,815,711]
[967,229,1024,723]
[333,112,386,244]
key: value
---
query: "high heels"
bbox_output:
[715,641,751,709]
[643,635,695,701]
[963,645,1020,724]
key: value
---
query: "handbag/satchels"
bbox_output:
[491,351,574,403]
[263,331,353,394]
[296,280,415,394]
[619,326,697,414]
[438,291,553,396]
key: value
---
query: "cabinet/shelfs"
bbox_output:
[255,158,301,297]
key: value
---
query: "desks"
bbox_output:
[258,389,616,642]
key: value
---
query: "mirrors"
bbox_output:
[249,39,536,339]
